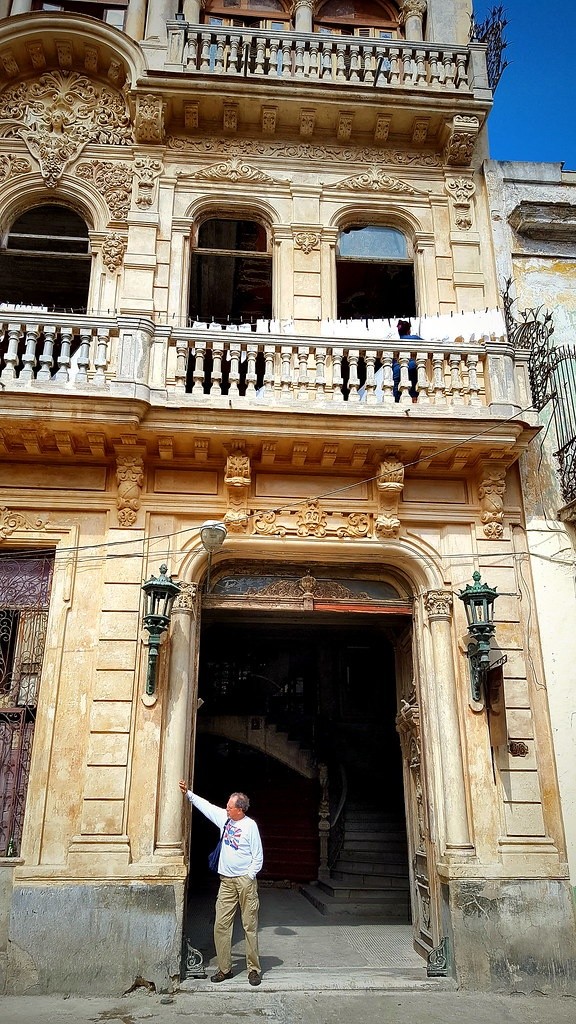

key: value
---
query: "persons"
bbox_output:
[179,778,263,986]
[390,320,424,401]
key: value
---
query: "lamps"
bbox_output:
[140,564,181,695]
[200,520,227,592]
[458,571,508,701]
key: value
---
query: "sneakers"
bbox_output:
[211,970,234,982]
[248,970,260,986]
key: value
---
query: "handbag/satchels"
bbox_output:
[209,816,231,872]
